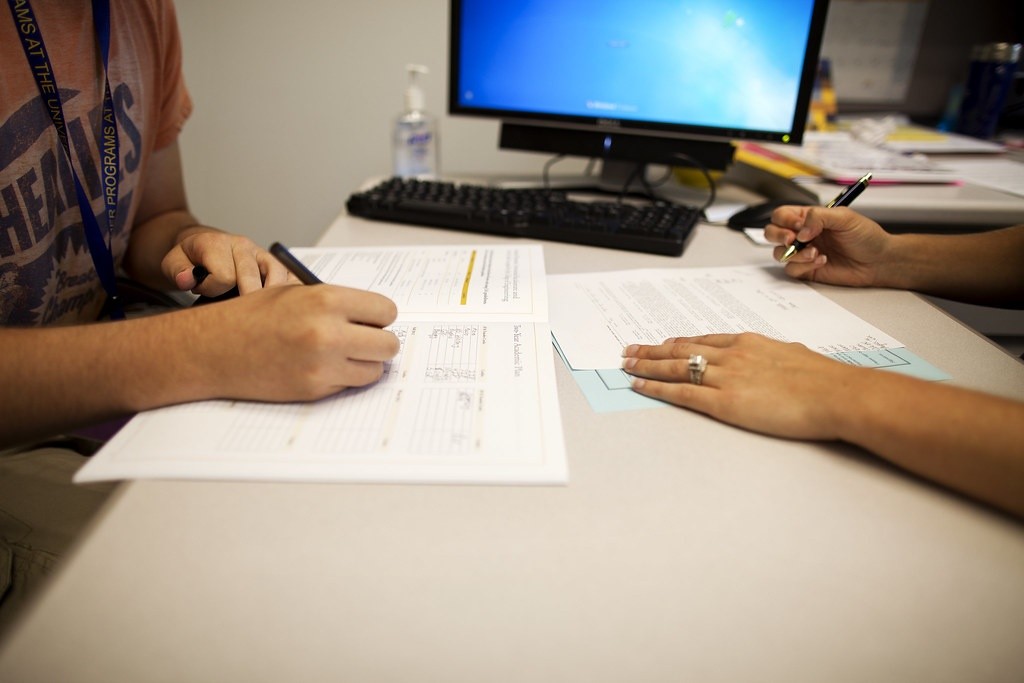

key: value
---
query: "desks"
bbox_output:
[0,154,1024,683]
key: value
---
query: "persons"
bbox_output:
[0,0,401,643]
[621,207,1023,521]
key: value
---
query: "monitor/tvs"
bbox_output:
[445,0,831,196]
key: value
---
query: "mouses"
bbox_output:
[727,199,815,231]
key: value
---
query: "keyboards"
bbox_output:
[344,176,707,256]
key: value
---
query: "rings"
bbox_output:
[688,354,709,384]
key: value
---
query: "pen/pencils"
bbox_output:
[778,173,874,262]
[269,243,321,286]
